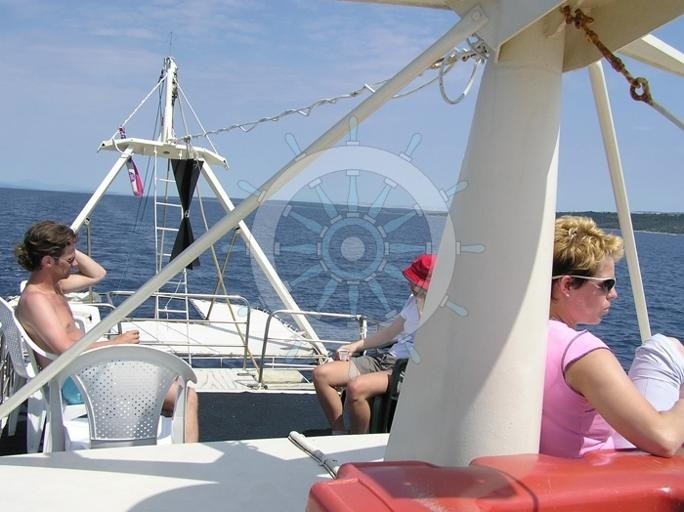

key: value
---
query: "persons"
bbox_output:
[538,214,684,460]
[313,253,438,436]
[16,221,200,444]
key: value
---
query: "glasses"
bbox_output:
[552,274,617,294]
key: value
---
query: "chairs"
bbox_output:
[0,298,199,453]
[338,340,410,433]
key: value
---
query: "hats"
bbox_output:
[401,253,437,290]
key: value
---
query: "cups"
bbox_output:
[339,351,349,361]
